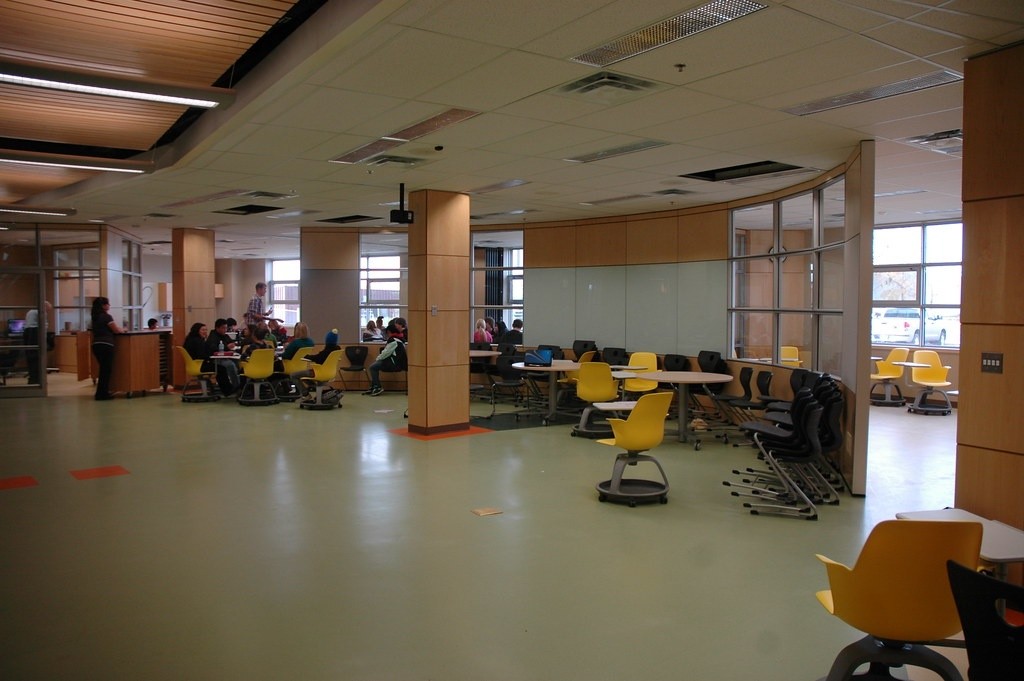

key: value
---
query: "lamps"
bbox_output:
[0,148,157,175]
[0,58,236,111]
[0,205,77,218]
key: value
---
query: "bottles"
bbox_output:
[219,340,224,355]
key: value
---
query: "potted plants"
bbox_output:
[60,327,72,334]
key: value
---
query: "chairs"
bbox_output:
[812,522,984,681]
[174,345,372,410]
[47,332,60,373]
[869,347,910,406]
[908,350,954,416]
[0,351,20,385]
[945,558,1024,681]
[378,347,408,396]
[470,342,848,521]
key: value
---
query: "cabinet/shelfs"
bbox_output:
[215,283,225,298]
[59,271,99,311]
[158,282,173,312]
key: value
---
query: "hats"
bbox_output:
[325,329,339,344]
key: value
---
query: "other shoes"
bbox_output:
[370,387,384,396]
[315,395,329,400]
[95,393,114,400]
[295,394,312,403]
[225,384,241,397]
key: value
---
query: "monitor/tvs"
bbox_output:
[8,319,25,334]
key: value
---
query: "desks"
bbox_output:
[510,359,580,426]
[636,371,734,451]
[210,355,242,373]
[609,365,647,400]
[611,371,638,380]
[891,361,931,369]
[897,507,1024,622]
[592,401,637,411]
[871,356,883,361]
[469,349,502,357]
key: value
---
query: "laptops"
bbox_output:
[524,350,552,367]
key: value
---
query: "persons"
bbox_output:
[366,325,407,395]
[207,313,314,393]
[364,317,407,340]
[485,317,496,337]
[23,301,52,384]
[183,323,240,397]
[247,282,273,332]
[474,319,492,343]
[148,318,158,329]
[501,319,522,344]
[90,297,128,401]
[290,328,341,400]
[492,321,509,343]
